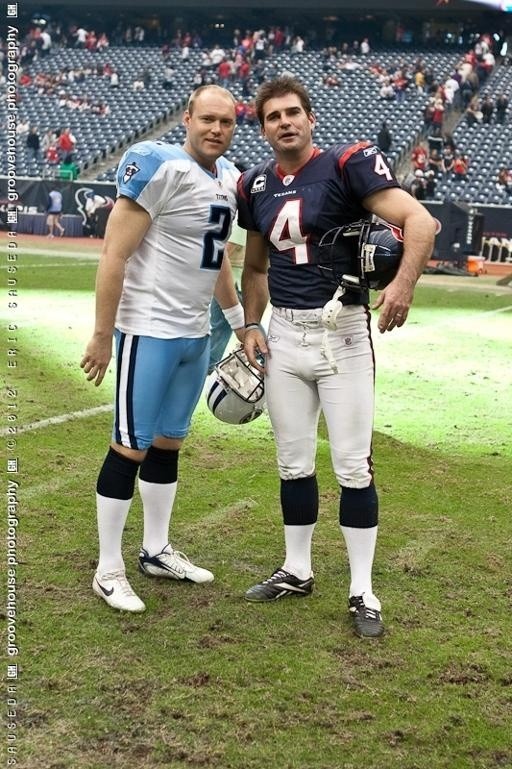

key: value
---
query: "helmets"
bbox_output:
[317,218,404,293]
[205,342,266,424]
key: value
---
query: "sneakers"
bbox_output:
[348,592,384,639]
[244,568,315,602]
[93,564,146,612]
[138,543,215,583]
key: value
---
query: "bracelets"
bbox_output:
[223,304,245,331]
[244,324,260,331]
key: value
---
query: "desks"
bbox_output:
[2,211,82,237]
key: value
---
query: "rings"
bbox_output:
[396,313,401,317]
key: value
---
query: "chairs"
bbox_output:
[2,33,510,209]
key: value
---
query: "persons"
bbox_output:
[235,77,438,637]
[0,11,512,235]
[78,83,249,614]
